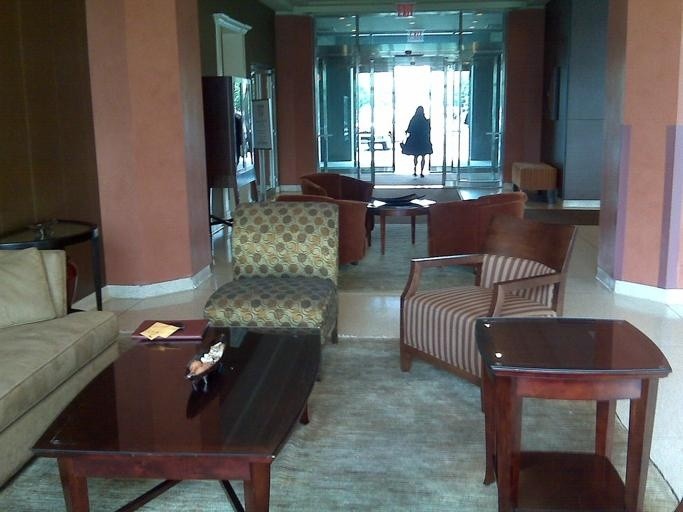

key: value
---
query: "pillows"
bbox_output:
[0,246,56,328]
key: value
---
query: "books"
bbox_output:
[133,319,211,342]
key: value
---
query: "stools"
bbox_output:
[510,162,557,204]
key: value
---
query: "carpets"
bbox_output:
[0,337,682,512]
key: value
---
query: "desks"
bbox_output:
[474,315,673,511]
[0,217,104,311]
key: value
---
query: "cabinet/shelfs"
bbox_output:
[200,75,256,228]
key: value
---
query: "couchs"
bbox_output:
[0,249,121,492]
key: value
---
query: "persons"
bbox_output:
[400,106,433,177]
